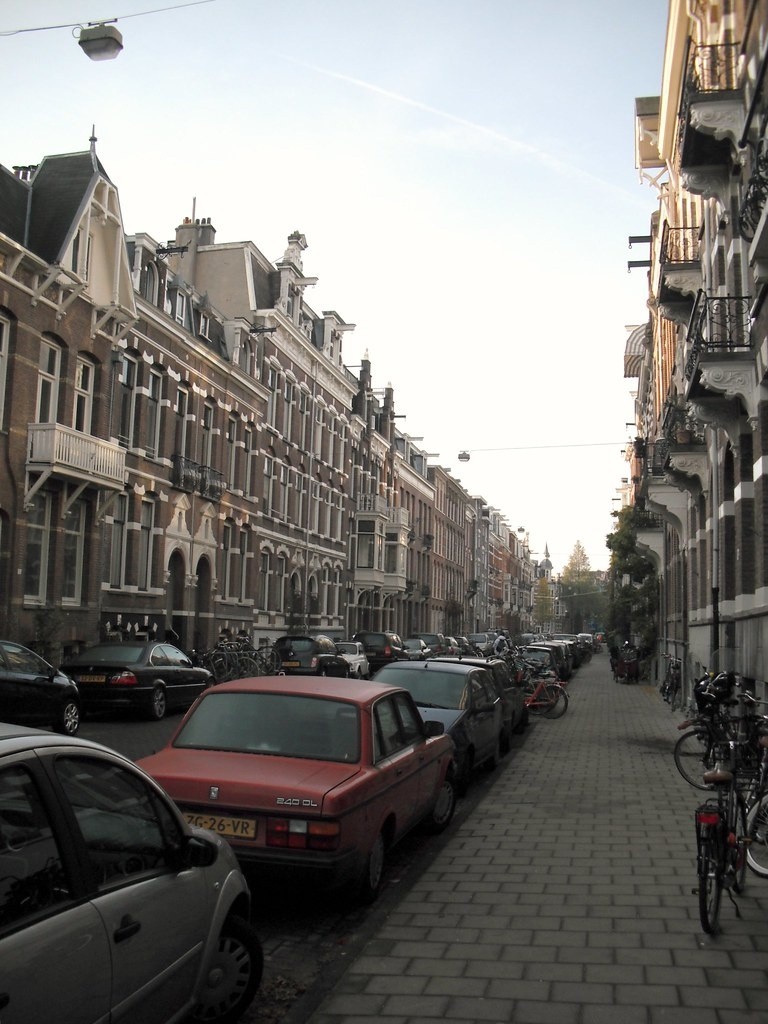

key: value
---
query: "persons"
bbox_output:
[492,632,508,655]
[609,644,620,671]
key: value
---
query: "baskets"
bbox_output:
[693,674,714,692]
[692,690,720,714]
[708,741,761,791]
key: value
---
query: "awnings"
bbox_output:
[624,323,646,378]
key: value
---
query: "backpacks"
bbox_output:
[496,639,506,652]
